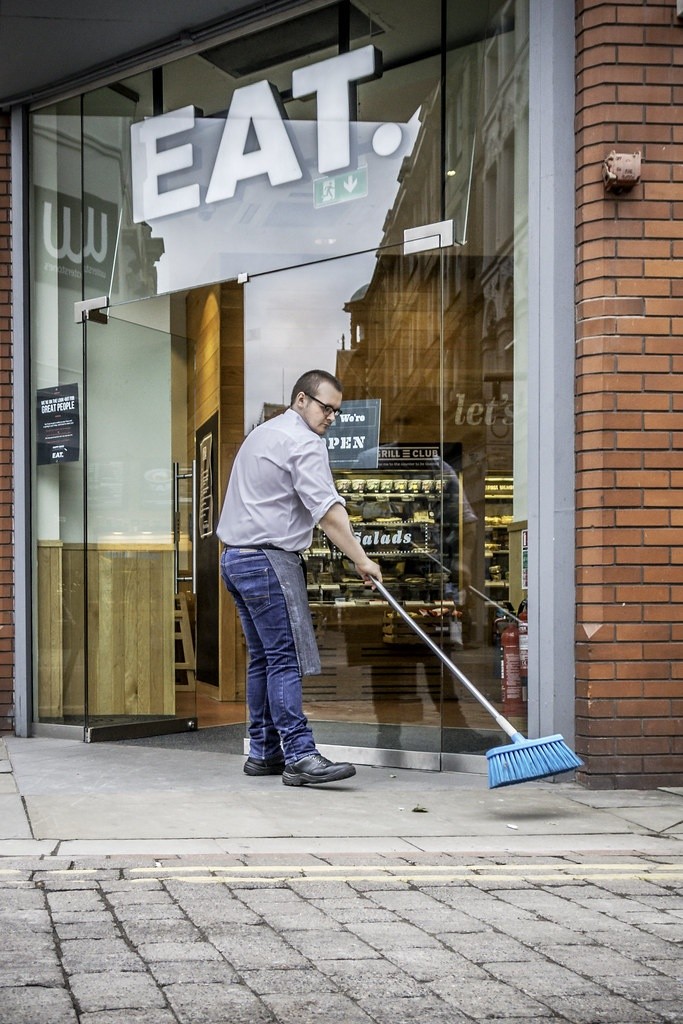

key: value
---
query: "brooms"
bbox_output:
[367,571,587,789]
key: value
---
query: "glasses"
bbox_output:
[304,392,342,417]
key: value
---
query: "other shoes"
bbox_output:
[376,731,402,750]
[441,727,502,754]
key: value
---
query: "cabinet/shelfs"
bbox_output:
[303,492,450,594]
[484,477,514,588]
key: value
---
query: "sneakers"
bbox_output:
[243,756,286,776]
[282,753,356,785]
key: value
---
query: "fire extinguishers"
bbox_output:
[495,602,528,716]
[517,598,528,677]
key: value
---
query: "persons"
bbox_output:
[340,401,502,769]
[215,370,381,788]
